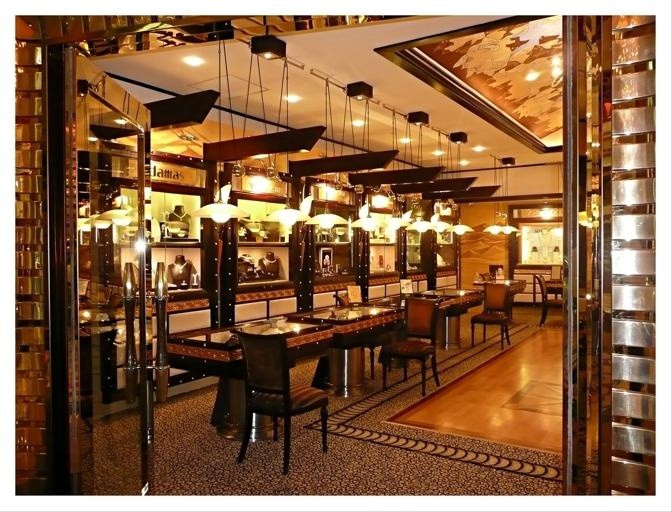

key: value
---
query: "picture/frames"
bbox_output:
[551,265,561,280]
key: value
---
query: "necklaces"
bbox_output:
[173,211,186,222]
[240,218,253,224]
[174,261,188,274]
[241,256,253,259]
[264,257,277,264]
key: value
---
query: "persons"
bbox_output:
[168,255,193,290]
[239,213,257,240]
[237,254,255,279]
[259,251,280,277]
[168,205,192,240]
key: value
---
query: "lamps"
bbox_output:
[405,118,437,233]
[251,35,287,61]
[303,77,349,230]
[262,59,311,228]
[351,99,387,233]
[432,131,452,233]
[380,110,408,232]
[407,111,429,126]
[502,157,516,165]
[347,81,373,102]
[449,132,468,144]
[193,35,250,224]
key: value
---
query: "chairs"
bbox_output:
[535,274,563,327]
[237,331,329,475]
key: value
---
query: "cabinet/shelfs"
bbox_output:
[100,142,459,405]
[513,273,563,307]
